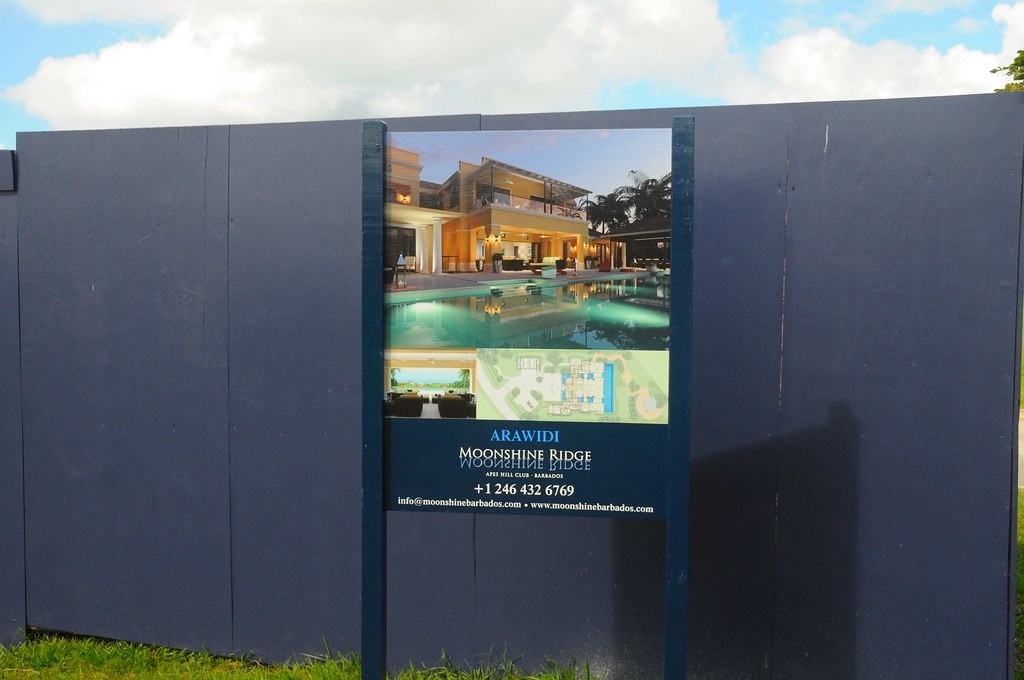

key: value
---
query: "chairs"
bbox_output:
[398,256,416,273]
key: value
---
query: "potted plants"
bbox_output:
[493,253,504,273]
